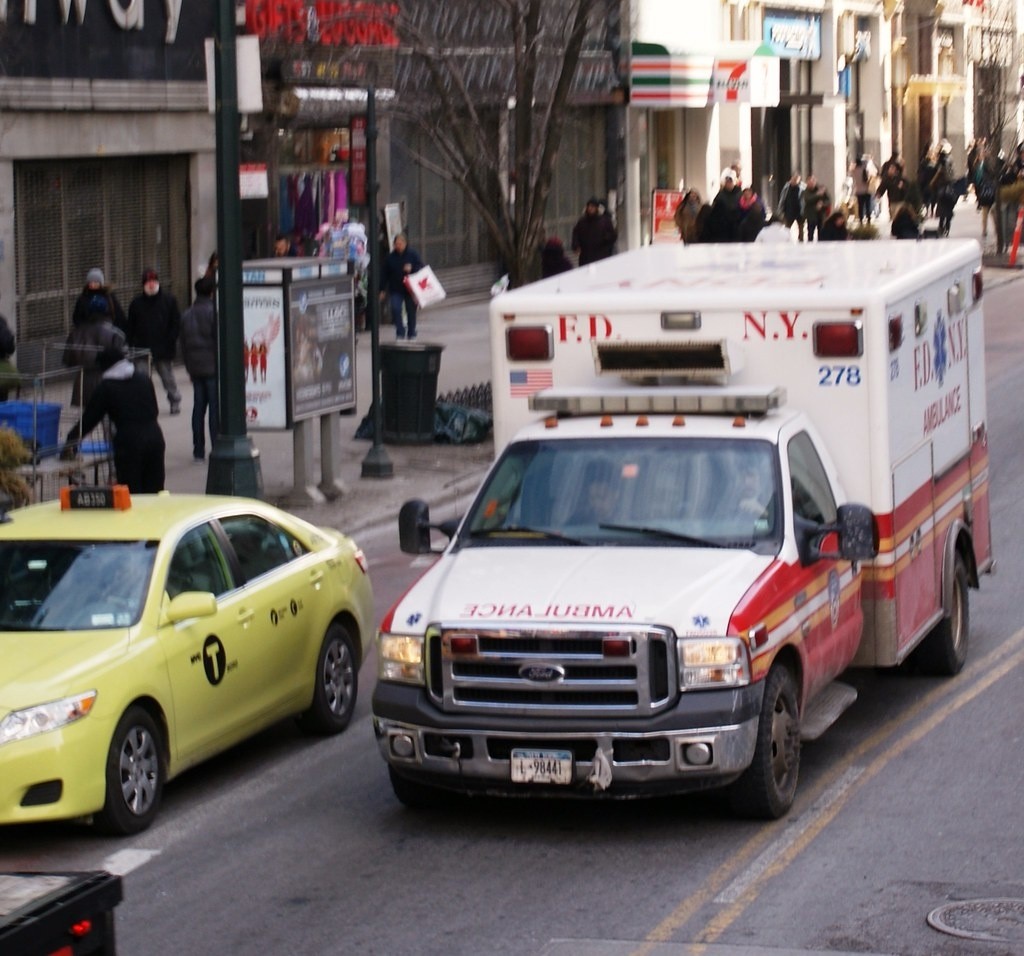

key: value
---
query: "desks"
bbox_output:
[17,451,115,505]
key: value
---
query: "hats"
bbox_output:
[142,269,160,285]
[86,268,105,288]
[587,196,600,206]
[550,237,563,246]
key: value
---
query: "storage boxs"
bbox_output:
[0,400,64,458]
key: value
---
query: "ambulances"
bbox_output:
[371,240,988,817]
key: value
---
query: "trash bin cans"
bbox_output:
[379,340,446,444]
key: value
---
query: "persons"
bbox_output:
[0,313,16,401]
[203,250,218,277]
[673,138,1024,243]
[63,266,181,416]
[182,277,218,460]
[60,345,166,494]
[257,228,319,259]
[94,550,179,607]
[542,196,619,278]
[565,459,636,526]
[381,232,424,340]
[718,450,806,520]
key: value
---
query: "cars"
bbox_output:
[0,480,371,831]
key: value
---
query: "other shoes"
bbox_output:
[195,458,207,465]
[170,402,180,414]
[396,336,416,341]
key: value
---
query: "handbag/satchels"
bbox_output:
[403,265,446,309]
[862,168,872,183]
[944,185,958,200]
[0,359,23,388]
